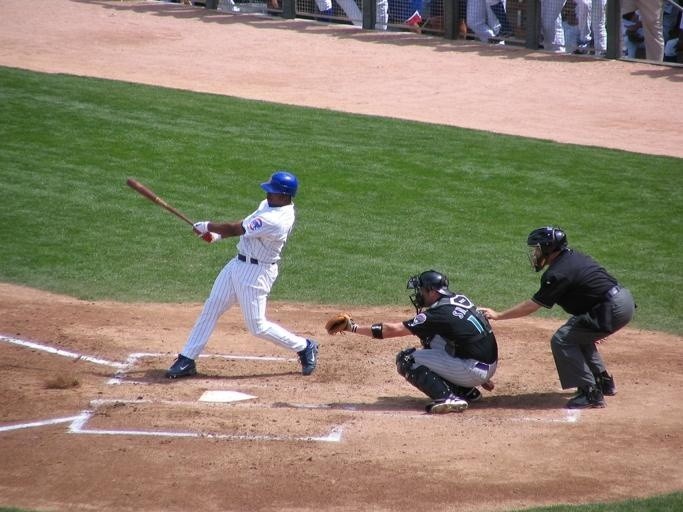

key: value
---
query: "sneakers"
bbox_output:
[297,339,319,376]
[166,354,197,378]
[425,387,482,414]
[217,4,241,15]
[567,375,617,409]
[573,40,593,55]
[488,25,514,44]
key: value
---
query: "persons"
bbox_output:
[476,227,634,408]
[165,172,318,380]
[326,270,498,415]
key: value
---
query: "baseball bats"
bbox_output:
[128,178,212,243]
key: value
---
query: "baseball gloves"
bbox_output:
[325,313,352,334]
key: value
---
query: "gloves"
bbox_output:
[191,221,221,244]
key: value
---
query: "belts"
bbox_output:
[599,283,624,303]
[238,254,275,264]
[475,363,489,370]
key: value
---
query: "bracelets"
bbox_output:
[349,324,359,333]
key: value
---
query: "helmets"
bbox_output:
[260,172,298,198]
[407,270,456,315]
[526,227,568,272]
